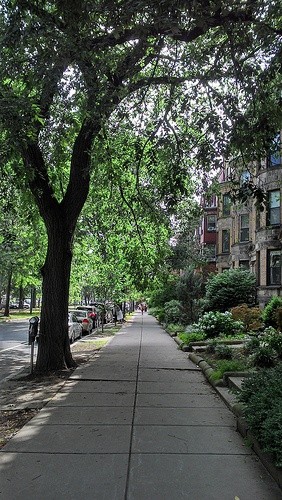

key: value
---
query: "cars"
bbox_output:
[76,305,100,329]
[68,313,83,343]
[70,310,94,335]
